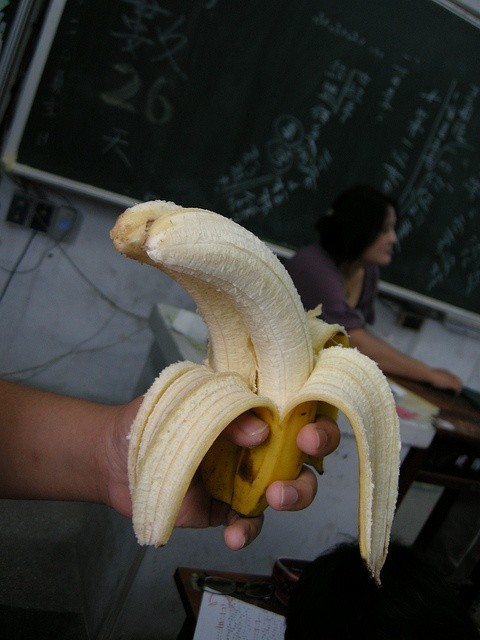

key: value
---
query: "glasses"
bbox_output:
[197,570,274,600]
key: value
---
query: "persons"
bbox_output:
[0,376,341,552]
[279,184,463,394]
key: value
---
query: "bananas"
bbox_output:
[107,198,324,508]
[131,205,402,588]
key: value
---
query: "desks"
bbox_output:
[173,564,303,639]
